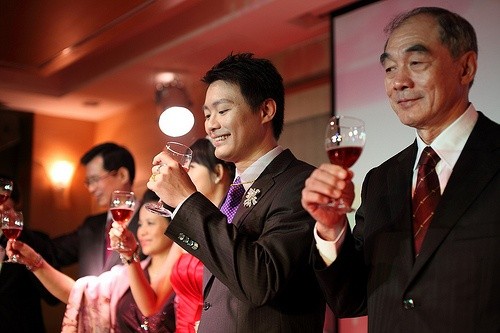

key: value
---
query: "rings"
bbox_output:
[156,165,163,174]
[152,174,158,181]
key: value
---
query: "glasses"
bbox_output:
[83,175,115,187]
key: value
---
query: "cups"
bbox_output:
[0,179,13,206]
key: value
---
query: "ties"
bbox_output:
[221,177,246,224]
[104,219,112,269]
[411,146,442,262]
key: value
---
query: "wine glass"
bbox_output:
[0,211,24,264]
[106,191,136,253]
[143,142,193,218]
[319,115,367,213]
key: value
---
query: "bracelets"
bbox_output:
[120,243,140,264]
[26,254,43,270]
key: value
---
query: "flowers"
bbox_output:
[244,187,260,207]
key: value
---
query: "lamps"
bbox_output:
[154,75,195,138]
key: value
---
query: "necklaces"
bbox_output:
[229,180,256,185]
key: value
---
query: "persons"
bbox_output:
[147,50,326,333]
[0,137,235,333]
[301,6,500,333]
